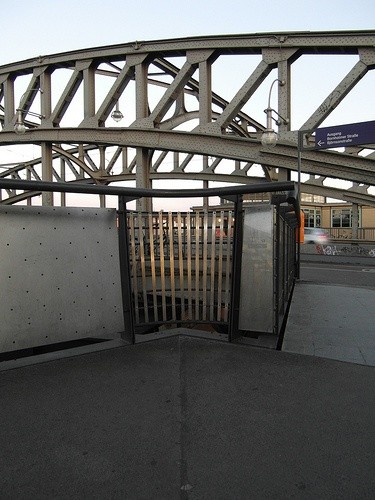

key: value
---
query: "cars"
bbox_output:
[304,228,328,245]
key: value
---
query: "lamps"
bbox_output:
[12,88,47,135]
[261,78,290,151]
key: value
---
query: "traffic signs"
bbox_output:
[298,121,375,152]
